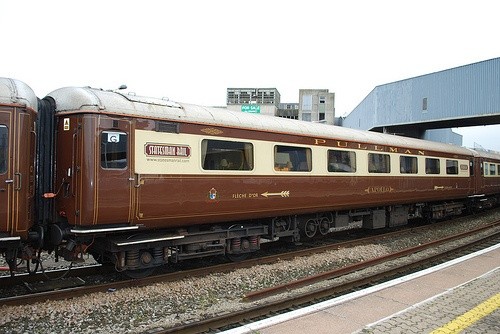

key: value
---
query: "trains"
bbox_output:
[1,76,499,280]
